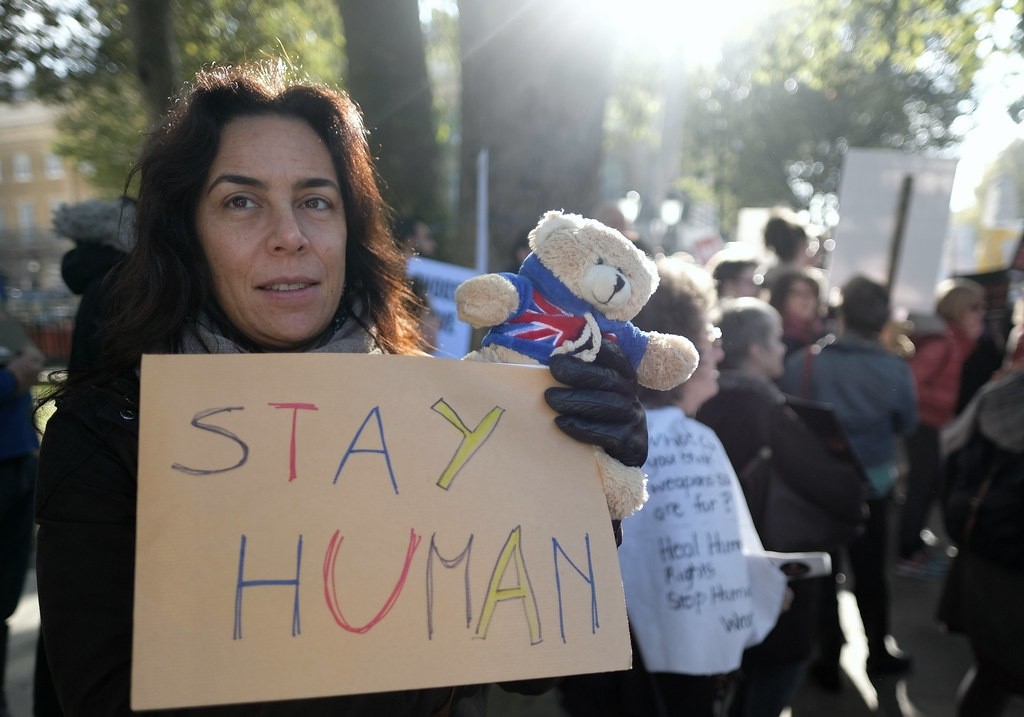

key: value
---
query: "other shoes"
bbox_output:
[868,652,910,677]
[806,667,841,696]
[897,556,935,578]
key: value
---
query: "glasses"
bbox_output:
[740,274,764,285]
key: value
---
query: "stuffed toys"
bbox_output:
[452,208,700,523]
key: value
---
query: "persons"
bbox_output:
[394,214,436,259]
[28,56,648,717]
[0,357,38,717]
[617,249,792,717]
[713,207,1024,717]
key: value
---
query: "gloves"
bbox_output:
[545,349,648,467]
